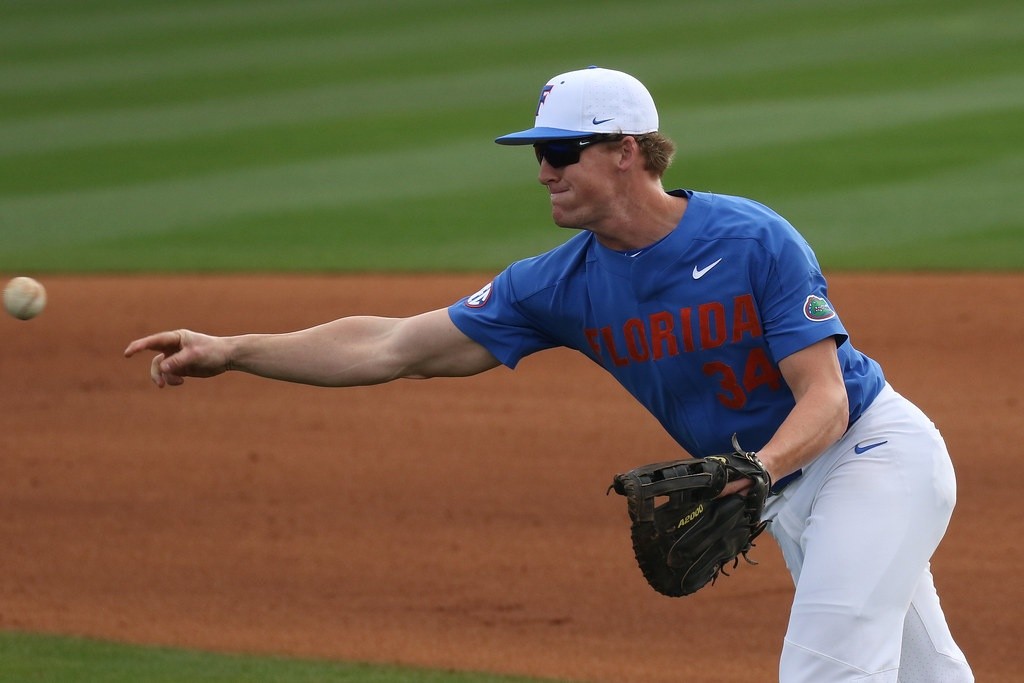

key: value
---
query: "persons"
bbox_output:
[125,67,981,682]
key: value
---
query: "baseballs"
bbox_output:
[3,277,48,321]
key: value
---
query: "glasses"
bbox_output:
[531,137,605,169]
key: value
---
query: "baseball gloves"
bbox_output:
[613,451,773,599]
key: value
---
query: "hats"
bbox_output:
[493,64,660,145]
